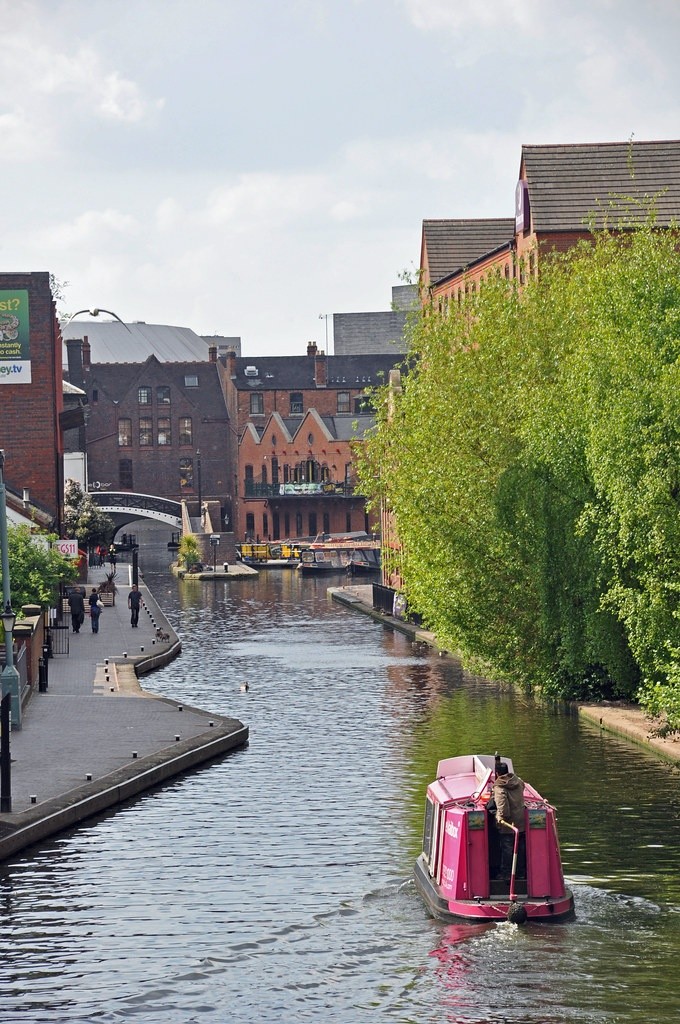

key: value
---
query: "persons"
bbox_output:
[492,762,526,881]
[89,587,102,633]
[109,545,117,573]
[68,587,85,632]
[128,584,143,628]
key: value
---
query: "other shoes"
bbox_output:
[135,625,138,627]
[73,628,77,631]
[516,875,525,878]
[76,630,79,632]
[132,625,135,627]
[497,874,510,880]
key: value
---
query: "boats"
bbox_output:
[166,531,183,551]
[113,531,140,554]
[239,531,307,566]
[288,531,381,577]
[414,753,579,929]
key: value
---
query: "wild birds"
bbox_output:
[240,681,249,693]
[155,626,170,644]
[411,640,448,657]
[57,308,132,339]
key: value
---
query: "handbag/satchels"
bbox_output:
[96,594,104,608]
[78,613,84,624]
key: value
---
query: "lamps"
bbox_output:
[0,600,16,632]
[38,657,46,665]
[42,644,49,653]
